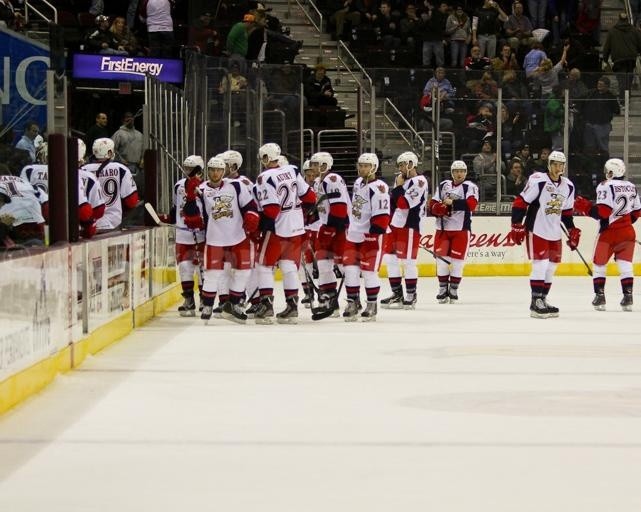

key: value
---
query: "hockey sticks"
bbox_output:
[311,273,347,320]
[192,229,205,283]
[304,192,341,225]
[306,240,319,280]
[561,223,592,275]
[143,202,206,234]
[150,132,203,196]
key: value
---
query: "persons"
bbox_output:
[0,0,338,240]
[574,158,641,312]
[315,0,621,198]
[174,143,391,318]
[374,151,430,307]
[602,13,641,106]
[511,151,583,315]
[427,160,480,300]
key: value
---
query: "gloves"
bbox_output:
[511,223,526,245]
[574,196,591,215]
[431,202,447,216]
[567,228,580,251]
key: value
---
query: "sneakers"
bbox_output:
[178,284,458,319]
[592,293,606,306]
[530,296,549,314]
[620,295,633,305]
[543,296,558,313]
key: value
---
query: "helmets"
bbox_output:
[358,152,379,175]
[450,160,468,182]
[184,150,243,178]
[604,158,626,180]
[35,142,48,165]
[78,137,115,159]
[257,143,288,167]
[396,151,418,170]
[547,150,566,174]
[303,151,333,173]
